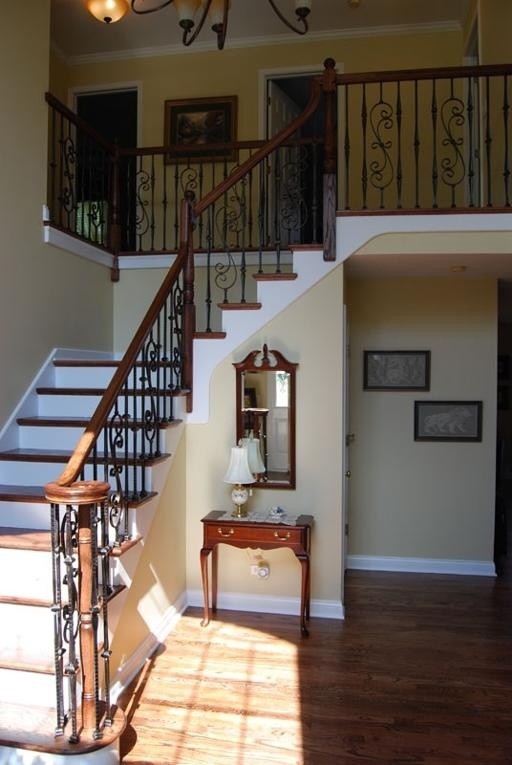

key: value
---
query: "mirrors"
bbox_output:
[232,344,298,491]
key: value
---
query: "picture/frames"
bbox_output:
[362,350,482,442]
[163,95,238,163]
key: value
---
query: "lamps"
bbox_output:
[224,444,257,518]
[242,429,266,482]
[131,0,311,48]
[85,1,130,25]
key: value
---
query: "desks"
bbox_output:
[200,510,314,638]
